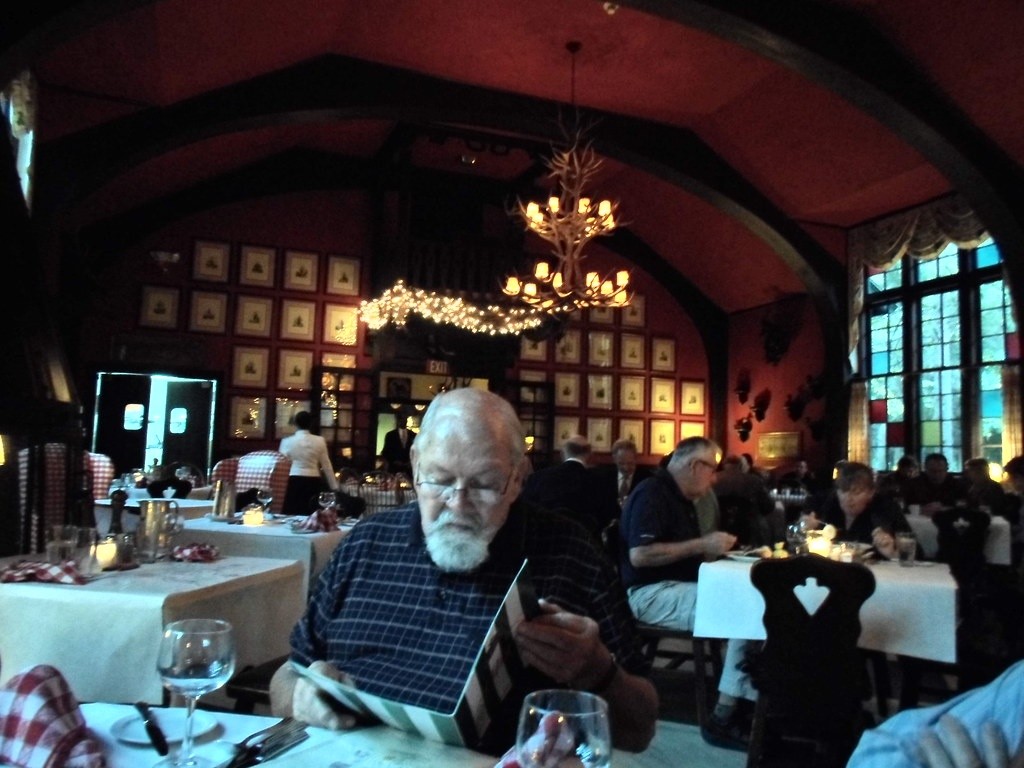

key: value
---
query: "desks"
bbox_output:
[692,542,960,753]
[908,514,1011,565]
[80,704,499,768]
[94,496,214,534]
[182,511,359,600]
[0,546,304,705]
[769,488,807,538]
[108,483,215,499]
[331,471,414,518]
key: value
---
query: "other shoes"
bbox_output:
[702,711,753,751]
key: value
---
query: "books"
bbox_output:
[287,558,538,748]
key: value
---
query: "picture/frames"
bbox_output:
[138,237,370,445]
[754,431,803,467]
[515,293,707,456]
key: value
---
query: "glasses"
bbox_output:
[694,459,719,475]
[413,458,518,505]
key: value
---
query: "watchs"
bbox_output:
[588,652,620,694]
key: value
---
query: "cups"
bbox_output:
[896,533,916,567]
[909,505,919,516]
[121,474,135,490]
[516,690,611,768]
[46,526,98,576]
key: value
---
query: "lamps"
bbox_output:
[500,35,635,310]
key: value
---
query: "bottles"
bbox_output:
[104,535,118,570]
[120,536,134,568]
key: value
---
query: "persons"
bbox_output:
[381,413,416,479]
[845,659,1024,768]
[267,387,660,753]
[526,435,1024,754]
[278,411,339,516]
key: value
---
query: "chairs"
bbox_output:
[0,446,1024,768]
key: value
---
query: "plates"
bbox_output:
[110,708,219,743]
[726,551,761,562]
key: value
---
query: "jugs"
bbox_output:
[211,480,237,519]
[136,500,179,561]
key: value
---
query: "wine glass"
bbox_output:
[258,487,272,515]
[153,620,235,767]
[319,493,335,510]
[785,523,808,557]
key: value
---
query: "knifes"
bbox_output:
[136,702,169,755]
